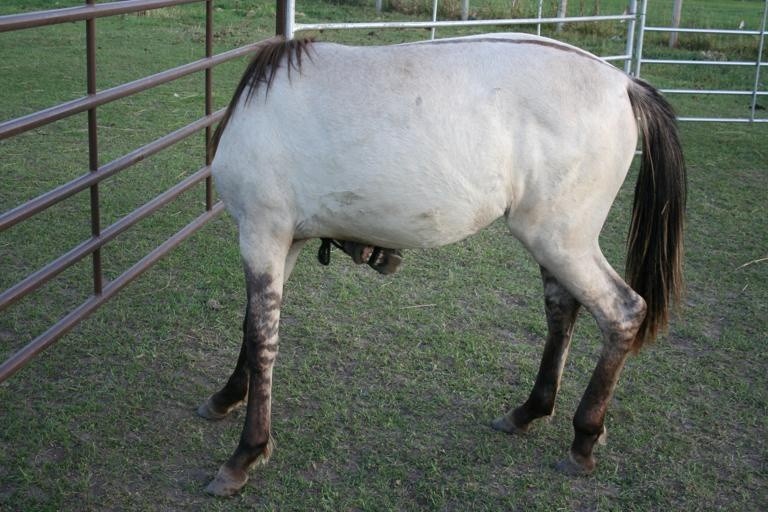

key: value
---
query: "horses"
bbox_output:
[194,30,690,500]
